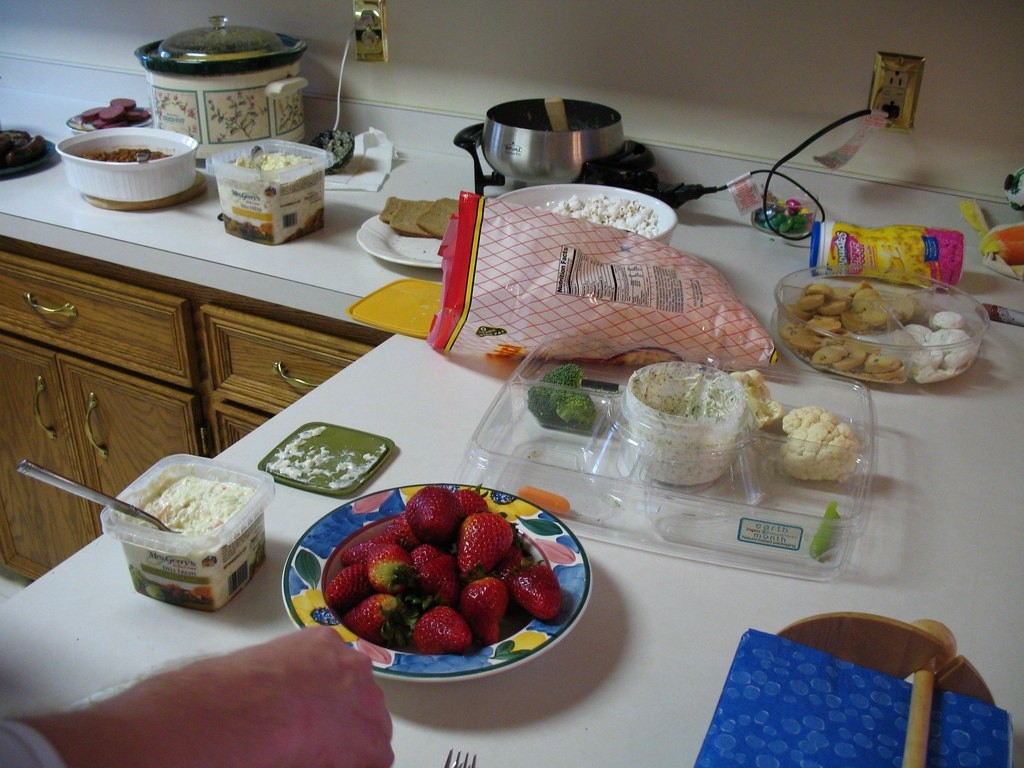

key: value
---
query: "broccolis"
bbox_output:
[527,364,598,431]
[731,370,861,481]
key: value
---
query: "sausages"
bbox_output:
[0,129,46,169]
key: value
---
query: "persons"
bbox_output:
[0,625,394,767]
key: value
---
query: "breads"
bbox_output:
[378,196,460,239]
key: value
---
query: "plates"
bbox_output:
[281,483,593,682]
[357,214,442,267]
[0,140,55,176]
[66,115,152,134]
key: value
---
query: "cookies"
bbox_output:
[779,282,921,384]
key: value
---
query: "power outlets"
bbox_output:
[353,0,389,62]
[864,49,926,136]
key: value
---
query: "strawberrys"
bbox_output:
[326,488,561,653]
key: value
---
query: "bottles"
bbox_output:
[810,221,966,290]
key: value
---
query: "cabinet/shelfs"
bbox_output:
[0,235,373,584]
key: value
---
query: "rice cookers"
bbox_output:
[134,16,306,159]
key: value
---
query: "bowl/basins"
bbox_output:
[496,183,678,245]
[460,333,873,583]
[310,129,355,174]
[101,454,275,612]
[206,138,335,246]
[481,99,624,185]
[751,185,819,234]
[55,127,199,201]
[775,262,990,384]
